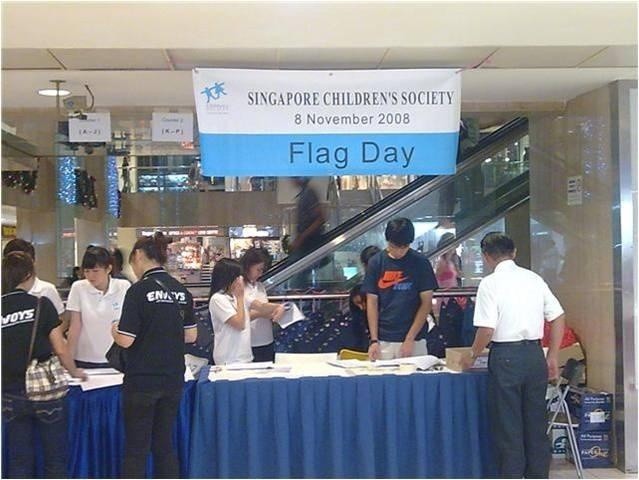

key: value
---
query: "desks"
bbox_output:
[2,353,498,479]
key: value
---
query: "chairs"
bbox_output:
[545,356,585,478]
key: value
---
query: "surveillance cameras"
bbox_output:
[63,95,87,110]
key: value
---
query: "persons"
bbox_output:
[452,120,482,211]
[289,176,326,289]
[122,156,132,192]
[189,160,201,189]
[456,232,566,477]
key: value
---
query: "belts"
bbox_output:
[491,340,541,347]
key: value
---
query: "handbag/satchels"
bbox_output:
[26,354,69,424]
[298,234,332,274]
[104,342,126,374]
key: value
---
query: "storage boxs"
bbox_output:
[552,384,615,468]
